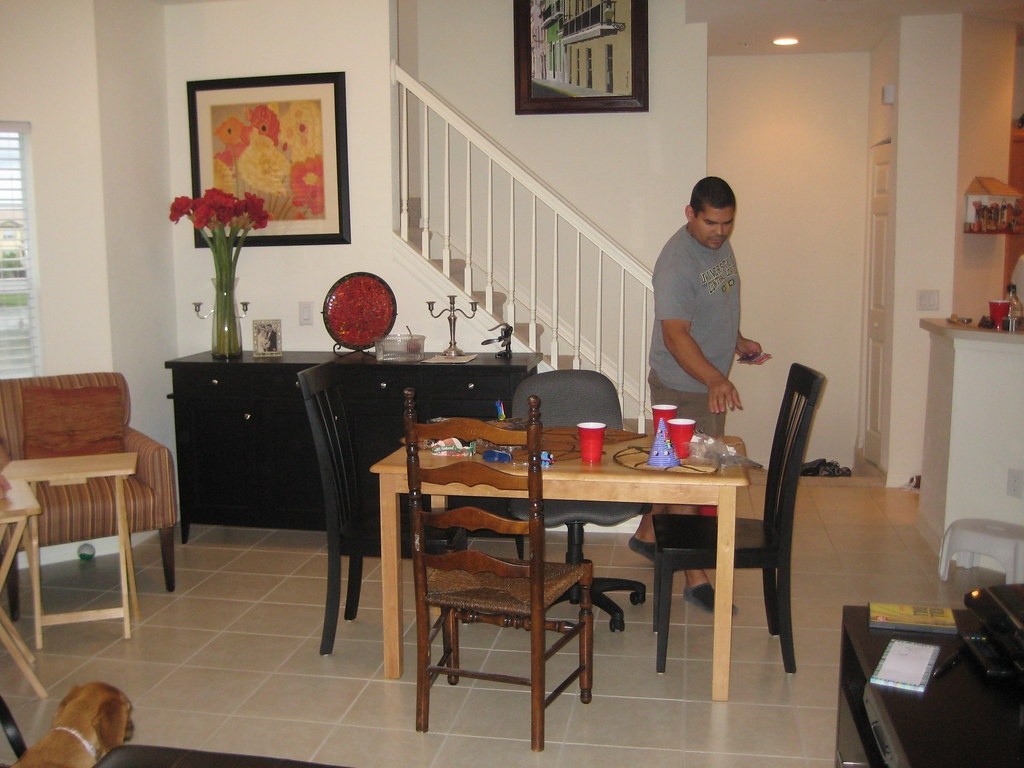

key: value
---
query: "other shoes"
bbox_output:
[683,582,737,616]
[628,534,655,561]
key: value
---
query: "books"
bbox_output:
[867,601,957,635]
[870,639,940,692]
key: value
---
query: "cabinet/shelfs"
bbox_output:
[163,348,541,547]
[833,605,1024,768]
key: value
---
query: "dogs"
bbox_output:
[10,680,135,768]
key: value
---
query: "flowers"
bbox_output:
[168,187,273,356]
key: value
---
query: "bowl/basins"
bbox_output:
[376,335,426,363]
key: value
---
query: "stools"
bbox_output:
[1,453,139,649]
[937,518,1024,586]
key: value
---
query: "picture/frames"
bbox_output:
[513,0,649,114]
[252,319,282,356]
[186,71,353,248]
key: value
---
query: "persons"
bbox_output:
[629,176,764,614]
[257,324,277,352]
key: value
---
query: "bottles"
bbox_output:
[1004,284,1022,326]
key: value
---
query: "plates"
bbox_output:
[946,317,972,323]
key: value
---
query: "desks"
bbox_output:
[0,451,38,701]
[369,426,751,700]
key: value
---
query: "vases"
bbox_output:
[209,276,242,358]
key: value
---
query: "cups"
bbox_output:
[1003,317,1018,331]
[577,422,606,464]
[667,418,696,459]
[652,404,678,435]
[989,300,1009,330]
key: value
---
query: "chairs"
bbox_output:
[507,366,646,633]
[295,359,468,659]
[0,371,180,622]
[403,386,594,751]
[651,362,826,676]
